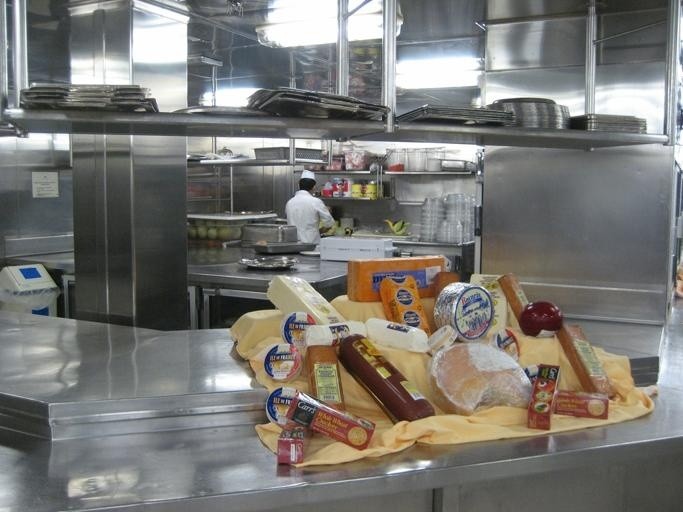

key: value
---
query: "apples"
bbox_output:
[520,301,562,336]
[189,248,230,264]
[188,219,242,239]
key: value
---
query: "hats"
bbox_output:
[301,170,315,180]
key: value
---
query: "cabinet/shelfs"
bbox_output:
[383,170,476,208]
[297,172,391,208]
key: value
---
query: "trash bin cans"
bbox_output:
[0,264,57,317]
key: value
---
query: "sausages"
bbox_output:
[339,334,434,422]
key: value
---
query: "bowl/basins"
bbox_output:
[420,193,473,244]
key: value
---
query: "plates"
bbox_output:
[237,256,299,269]
[18,81,152,112]
[397,97,648,134]
[247,86,391,121]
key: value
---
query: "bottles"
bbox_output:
[319,177,378,199]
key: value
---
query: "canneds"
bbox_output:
[366,184,376,198]
[352,184,362,197]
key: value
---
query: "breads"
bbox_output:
[429,343,532,416]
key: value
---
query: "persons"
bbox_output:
[284,169,336,245]
